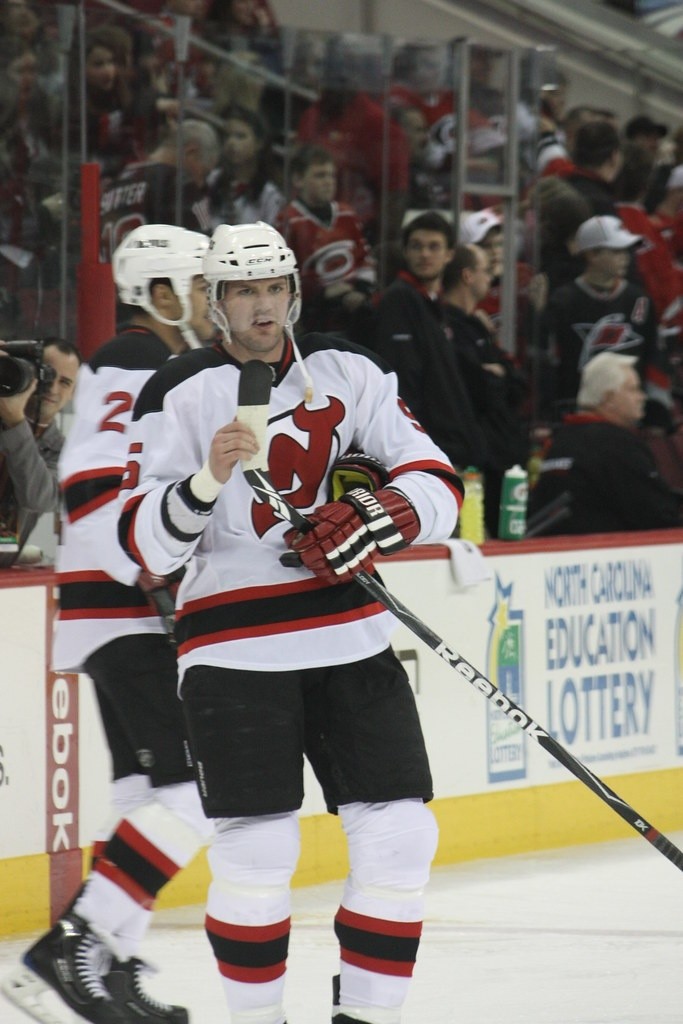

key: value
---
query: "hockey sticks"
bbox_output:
[235,356,682,869]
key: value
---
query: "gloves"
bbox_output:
[281,488,421,587]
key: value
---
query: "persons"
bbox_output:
[2,226,218,1024]
[108,221,465,1024]
[0,0,682,569]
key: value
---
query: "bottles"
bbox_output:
[459,466,484,546]
[498,465,528,540]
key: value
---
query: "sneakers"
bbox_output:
[92,939,189,1024]
[0,882,129,1024]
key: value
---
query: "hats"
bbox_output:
[460,210,503,245]
[572,216,642,255]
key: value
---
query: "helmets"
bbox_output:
[201,222,301,303]
[108,224,213,326]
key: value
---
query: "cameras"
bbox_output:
[0,341,55,397]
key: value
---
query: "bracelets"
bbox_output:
[189,462,228,504]
